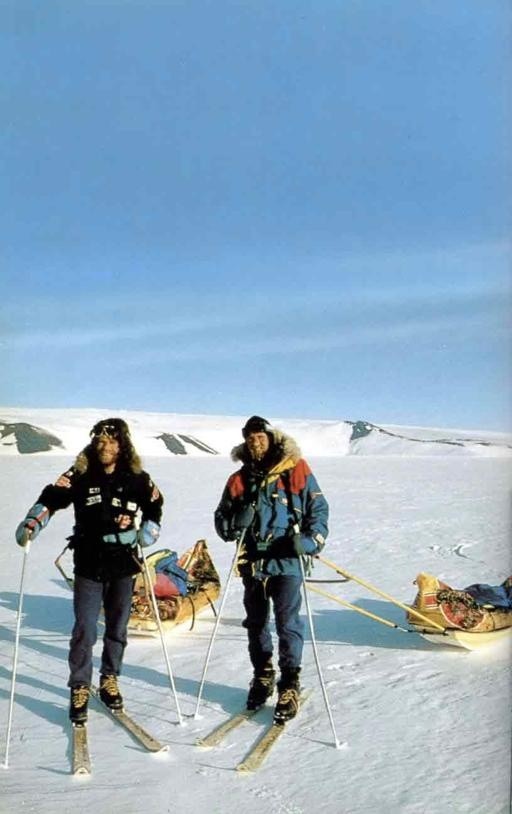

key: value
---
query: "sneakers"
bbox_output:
[69,687,88,723]
[100,676,123,709]
[275,685,299,720]
[249,671,275,709]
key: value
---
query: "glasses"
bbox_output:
[94,424,116,438]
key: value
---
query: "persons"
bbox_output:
[13,415,163,726]
[213,413,329,721]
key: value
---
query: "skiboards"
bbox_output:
[73,681,161,775]
[195,677,312,773]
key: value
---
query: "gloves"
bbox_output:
[16,504,49,546]
[137,520,161,546]
[234,508,255,528]
[293,535,316,556]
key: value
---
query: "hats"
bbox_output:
[242,416,273,438]
